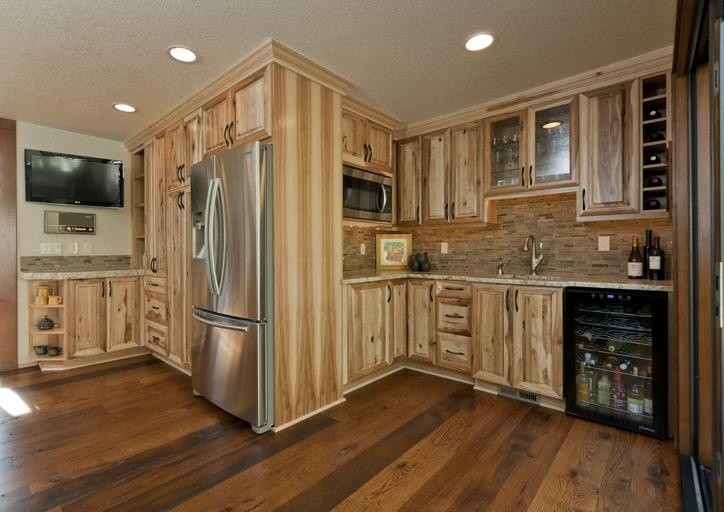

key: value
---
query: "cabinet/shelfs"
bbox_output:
[27,280,64,364]
[146,130,167,277]
[405,279,436,367]
[577,77,638,215]
[348,283,395,380]
[395,136,423,224]
[340,108,393,172]
[471,282,565,398]
[163,109,201,190]
[421,117,484,227]
[636,71,669,215]
[168,186,189,375]
[68,275,140,357]
[482,93,579,199]
[201,64,271,157]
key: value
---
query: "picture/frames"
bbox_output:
[374,232,413,271]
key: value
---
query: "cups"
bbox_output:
[36,295,48,304]
[48,296,61,304]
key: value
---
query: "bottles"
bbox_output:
[491,121,570,187]
[627,229,665,280]
[642,110,664,209]
[576,330,653,425]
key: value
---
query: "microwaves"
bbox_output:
[343,165,393,222]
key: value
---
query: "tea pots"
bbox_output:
[36,284,54,303]
[33,345,59,356]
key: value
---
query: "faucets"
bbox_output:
[523,235,544,276]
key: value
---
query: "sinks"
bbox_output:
[497,273,562,282]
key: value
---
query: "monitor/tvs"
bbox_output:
[23,147,126,210]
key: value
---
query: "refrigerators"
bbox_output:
[190,139,274,435]
